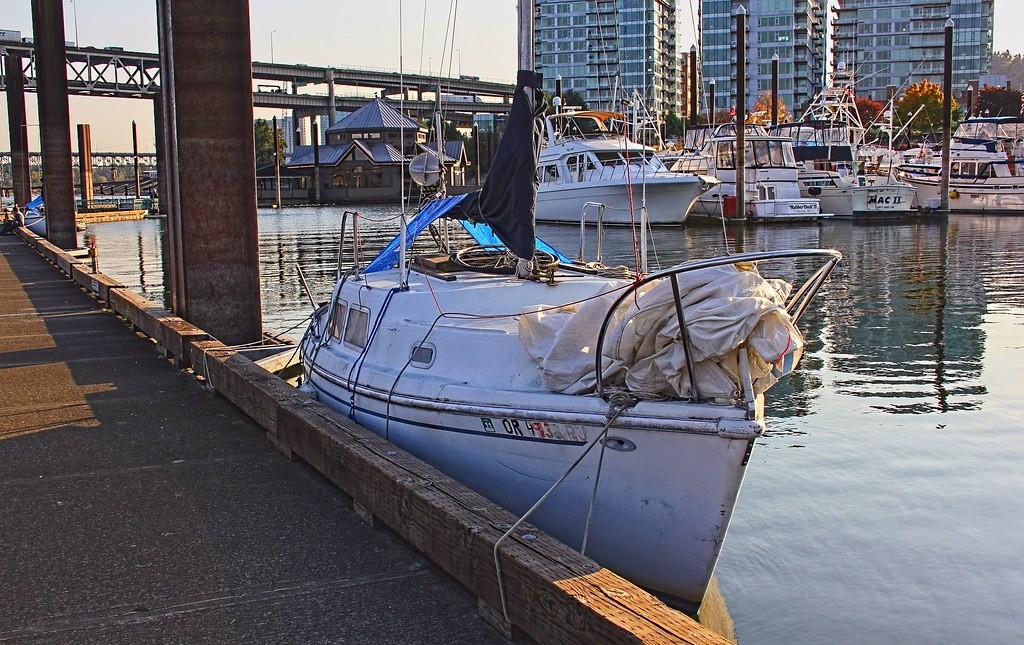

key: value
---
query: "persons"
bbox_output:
[0,207,24,236]
[858,160,866,175]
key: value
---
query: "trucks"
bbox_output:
[441,95,483,103]
[0,29,22,43]
[66,41,75,47]
[22,37,34,44]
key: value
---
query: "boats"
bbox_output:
[531,96,721,229]
[25,209,47,236]
[653,44,1024,222]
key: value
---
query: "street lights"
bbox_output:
[270,30,276,64]
[353,80,359,98]
[456,49,462,77]
[428,57,435,76]
[69,0,79,48]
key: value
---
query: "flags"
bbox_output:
[919,141,925,157]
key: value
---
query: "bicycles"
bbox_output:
[0,216,19,236]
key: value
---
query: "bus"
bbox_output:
[75,195,151,212]
[459,75,480,83]
[104,47,123,52]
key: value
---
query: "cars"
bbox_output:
[85,46,95,50]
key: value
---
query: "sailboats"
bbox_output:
[303,0,843,620]
